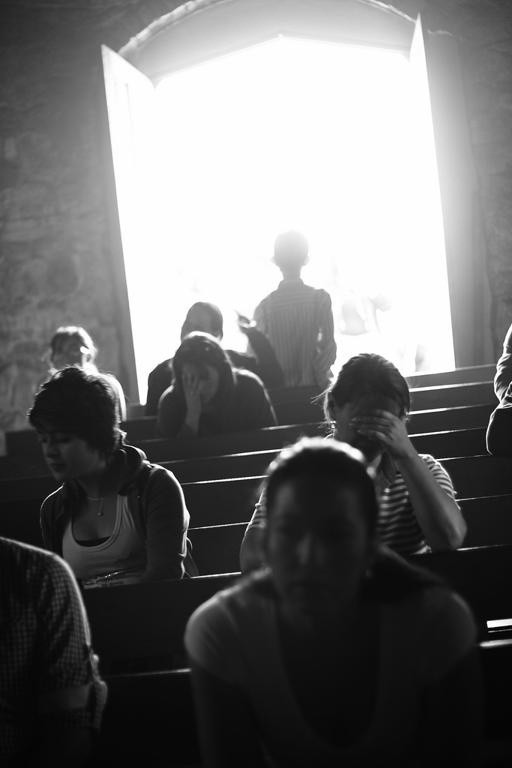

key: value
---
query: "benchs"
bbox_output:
[0,361,512,768]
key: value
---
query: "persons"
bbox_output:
[484,324,512,458]
[184,438,490,767]
[0,534,110,767]
[142,302,286,418]
[154,331,277,439]
[27,366,196,588]
[246,230,336,388]
[47,326,127,425]
[240,354,482,636]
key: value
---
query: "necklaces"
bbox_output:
[85,494,106,516]
